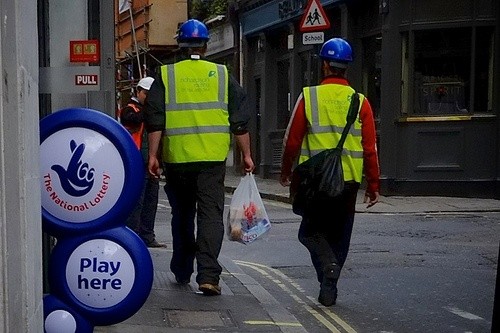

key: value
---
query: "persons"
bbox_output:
[279,38,380,306]
[120,18,256,294]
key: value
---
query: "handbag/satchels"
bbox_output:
[289,148,345,216]
[227,170,271,244]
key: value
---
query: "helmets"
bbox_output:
[136,77,155,91]
[319,38,352,61]
[173,19,210,44]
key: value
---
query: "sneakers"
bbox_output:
[176,277,190,284]
[147,239,167,248]
[199,283,222,296]
[318,262,340,307]
